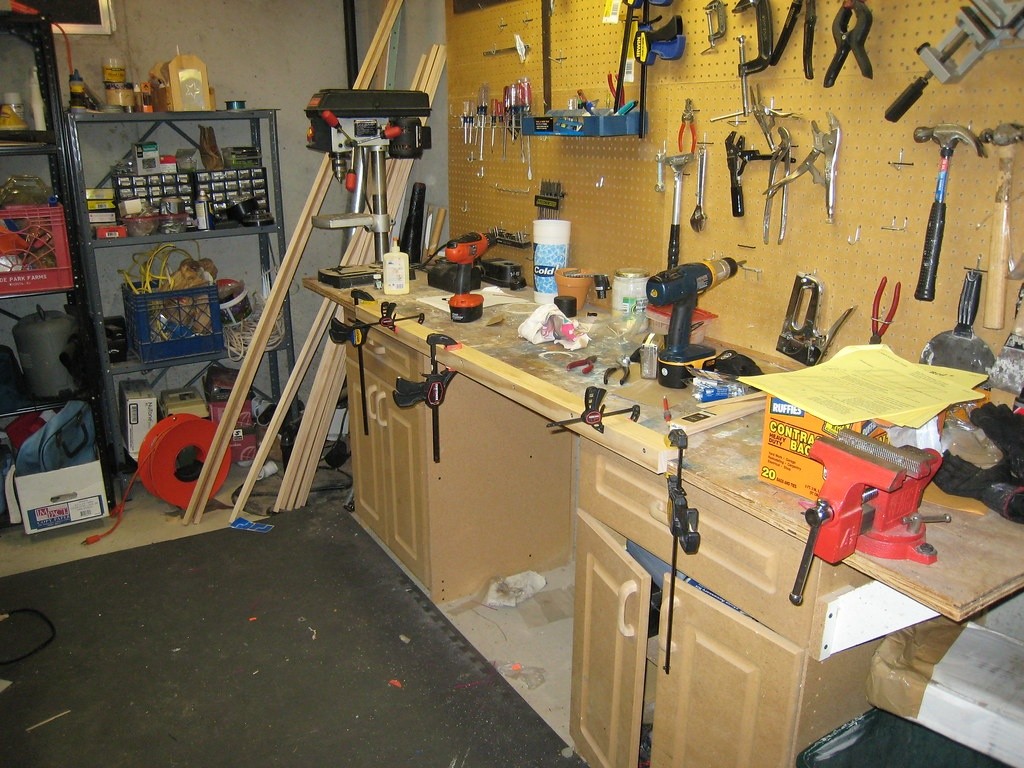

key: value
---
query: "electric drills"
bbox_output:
[646,257,747,389]
[445,232,497,323]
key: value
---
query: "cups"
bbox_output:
[641,345,658,379]
[532,219,571,302]
[554,267,592,309]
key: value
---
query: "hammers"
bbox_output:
[978,121,1023,329]
[914,125,988,301]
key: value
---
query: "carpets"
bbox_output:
[0,503,590,767]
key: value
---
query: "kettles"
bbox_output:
[159,194,184,214]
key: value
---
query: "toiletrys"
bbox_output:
[383,237,411,296]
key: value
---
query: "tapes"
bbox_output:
[225,100,246,110]
[226,197,258,220]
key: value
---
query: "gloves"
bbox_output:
[969,402,1024,478]
[931,449,1024,524]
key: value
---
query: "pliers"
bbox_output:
[868,277,901,344]
[678,99,697,153]
[762,112,840,223]
[725,131,796,216]
[566,355,597,373]
[762,127,792,245]
[608,73,625,107]
[604,355,630,386]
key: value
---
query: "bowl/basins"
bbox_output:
[225,100,246,111]
[219,288,256,327]
[159,214,188,234]
[223,196,258,220]
[120,215,161,236]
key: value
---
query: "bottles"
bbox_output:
[196,189,214,231]
[610,268,651,333]
[70,57,151,114]
[373,274,381,290]
[0,92,28,130]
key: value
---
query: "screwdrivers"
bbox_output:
[464,78,532,181]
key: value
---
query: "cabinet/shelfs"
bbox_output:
[61,108,301,504]
[0,11,117,515]
[302,276,1024,768]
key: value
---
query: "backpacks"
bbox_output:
[4,402,102,525]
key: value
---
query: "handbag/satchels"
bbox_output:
[0,410,58,454]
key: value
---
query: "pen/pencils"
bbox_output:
[663,396,672,424]
[614,99,639,116]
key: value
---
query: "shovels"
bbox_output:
[987,296,1024,396]
[918,270,996,390]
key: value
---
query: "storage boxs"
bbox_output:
[14,443,109,534]
[121,278,224,364]
[645,303,720,344]
[159,387,210,418]
[119,379,157,459]
[0,203,74,297]
[757,391,889,502]
